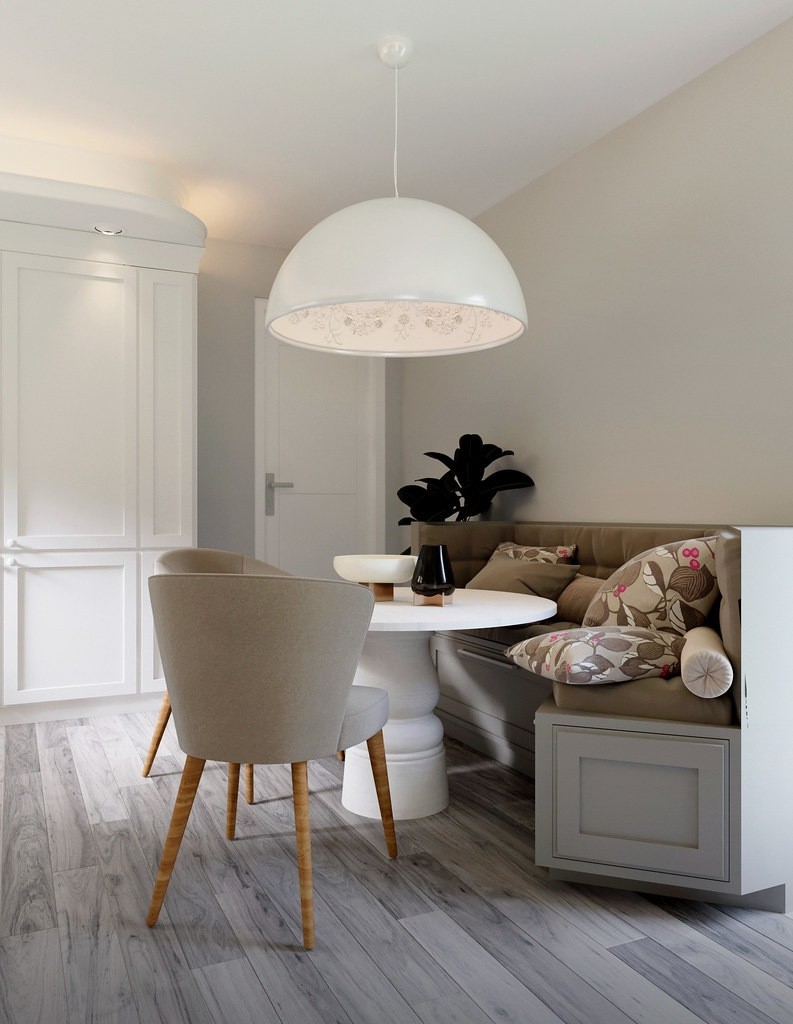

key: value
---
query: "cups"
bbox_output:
[411,544,455,597]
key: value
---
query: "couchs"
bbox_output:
[410,521,793,731]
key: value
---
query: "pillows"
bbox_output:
[555,574,609,625]
[486,539,577,572]
[682,628,733,700]
[582,536,720,637]
[464,550,580,599]
[504,626,686,685]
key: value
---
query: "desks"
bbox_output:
[340,586,558,821]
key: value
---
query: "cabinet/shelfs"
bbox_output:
[0,217,206,724]
[427,627,793,914]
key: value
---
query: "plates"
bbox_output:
[334,554,418,583]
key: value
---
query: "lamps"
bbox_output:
[264,36,529,361]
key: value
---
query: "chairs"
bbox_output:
[143,548,401,952]
